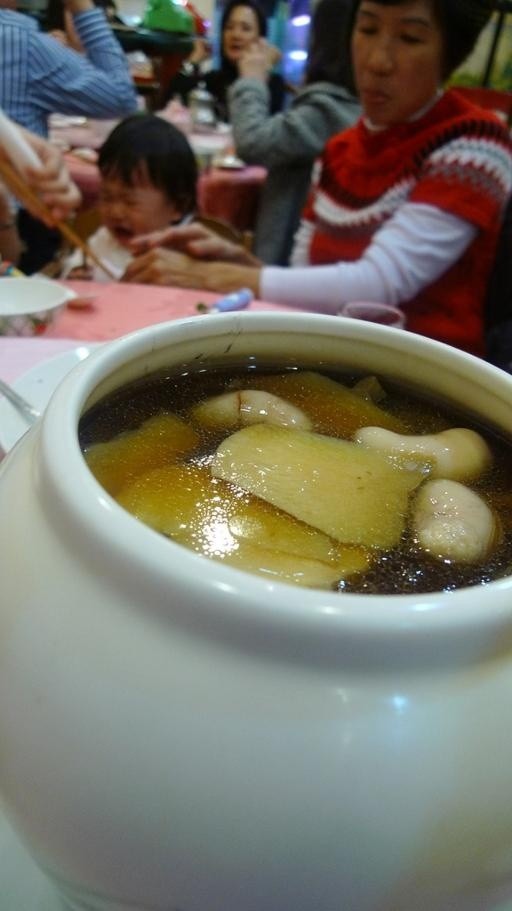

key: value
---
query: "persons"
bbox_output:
[1,0,511,359]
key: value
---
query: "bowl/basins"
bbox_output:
[0,275,73,337]
